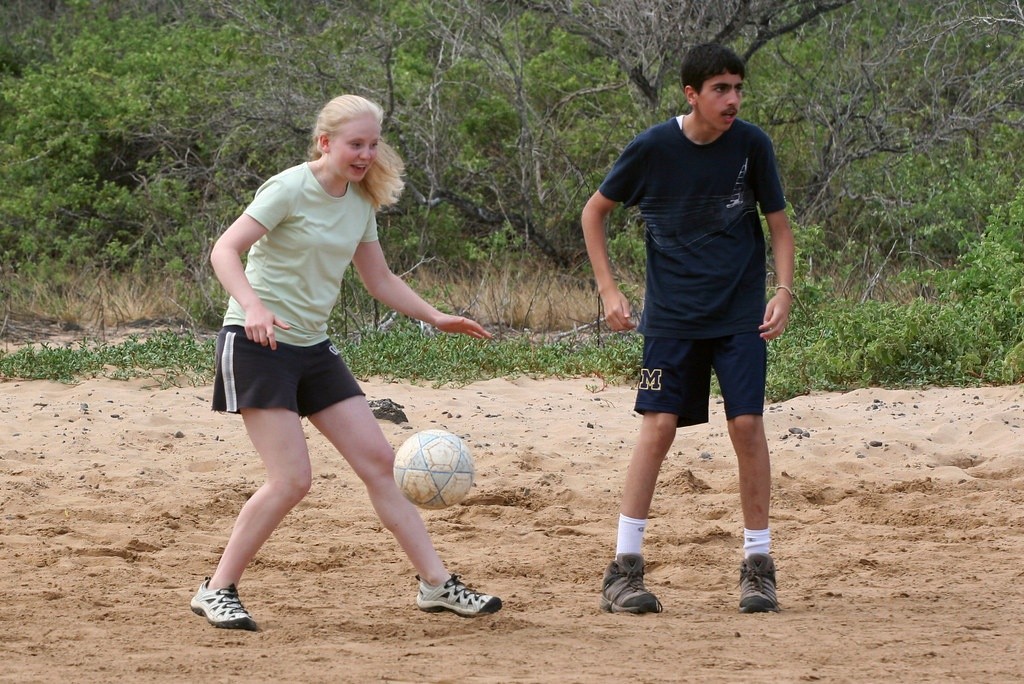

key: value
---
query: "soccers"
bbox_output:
[392,428,474,510]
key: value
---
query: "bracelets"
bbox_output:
[776,286,793,298]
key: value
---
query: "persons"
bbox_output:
[582,43,792,613]
[189,94,503,629]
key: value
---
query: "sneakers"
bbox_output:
[600,553,663,614]
[415,574,502,618]
[735,553,780,614]
[190,577,256,631]
